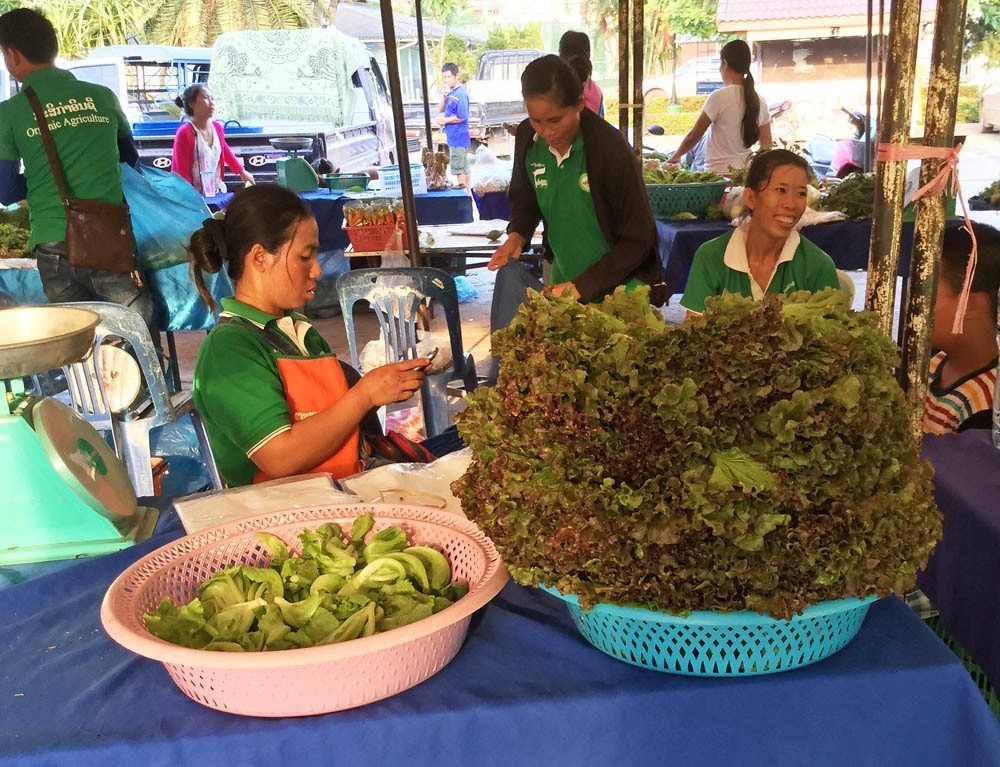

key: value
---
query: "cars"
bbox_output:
[641,54,757,106]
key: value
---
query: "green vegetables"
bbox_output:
[643,168,749,220]
[816,172,875,220]
[142,512,471,652]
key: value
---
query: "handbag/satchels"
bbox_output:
[65,199,135,272]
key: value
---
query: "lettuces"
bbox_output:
[450,282,943,618]
[0,208,37,258]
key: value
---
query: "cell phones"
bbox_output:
[412,347,438,371]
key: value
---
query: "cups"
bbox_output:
[200,171,217,197]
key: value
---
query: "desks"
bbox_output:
[919,429,998,694]
[196,188,511,280]
[0,261,233,394]
[656,215,977,293]
[0,532,1000,767]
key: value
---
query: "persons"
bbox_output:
[171,83,255,192]
[0,8,164,394]
[665,39,773,171]
[438,63,470,191]
[486,53,668,309]
[182,182,433,488]
[919,224,1000,434]
[504,32,603,288]
[679,150,839,322]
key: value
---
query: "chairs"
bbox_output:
[45,301,224,498]
[335,267,478,439]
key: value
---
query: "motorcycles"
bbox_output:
[798,105,877,190]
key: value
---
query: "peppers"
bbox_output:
[343,203,405,226]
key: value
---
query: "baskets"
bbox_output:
[100,502,512,717]
[321,172,371,194]
[340,215,410,252]
[538,582,895,677]
[377,163,428,197]
[644,178,732,221]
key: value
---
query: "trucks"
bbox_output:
[401,48,559,157]
[131,28,399,192]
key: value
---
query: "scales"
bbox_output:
[269,137,320,192]
[1,305,161,566]
